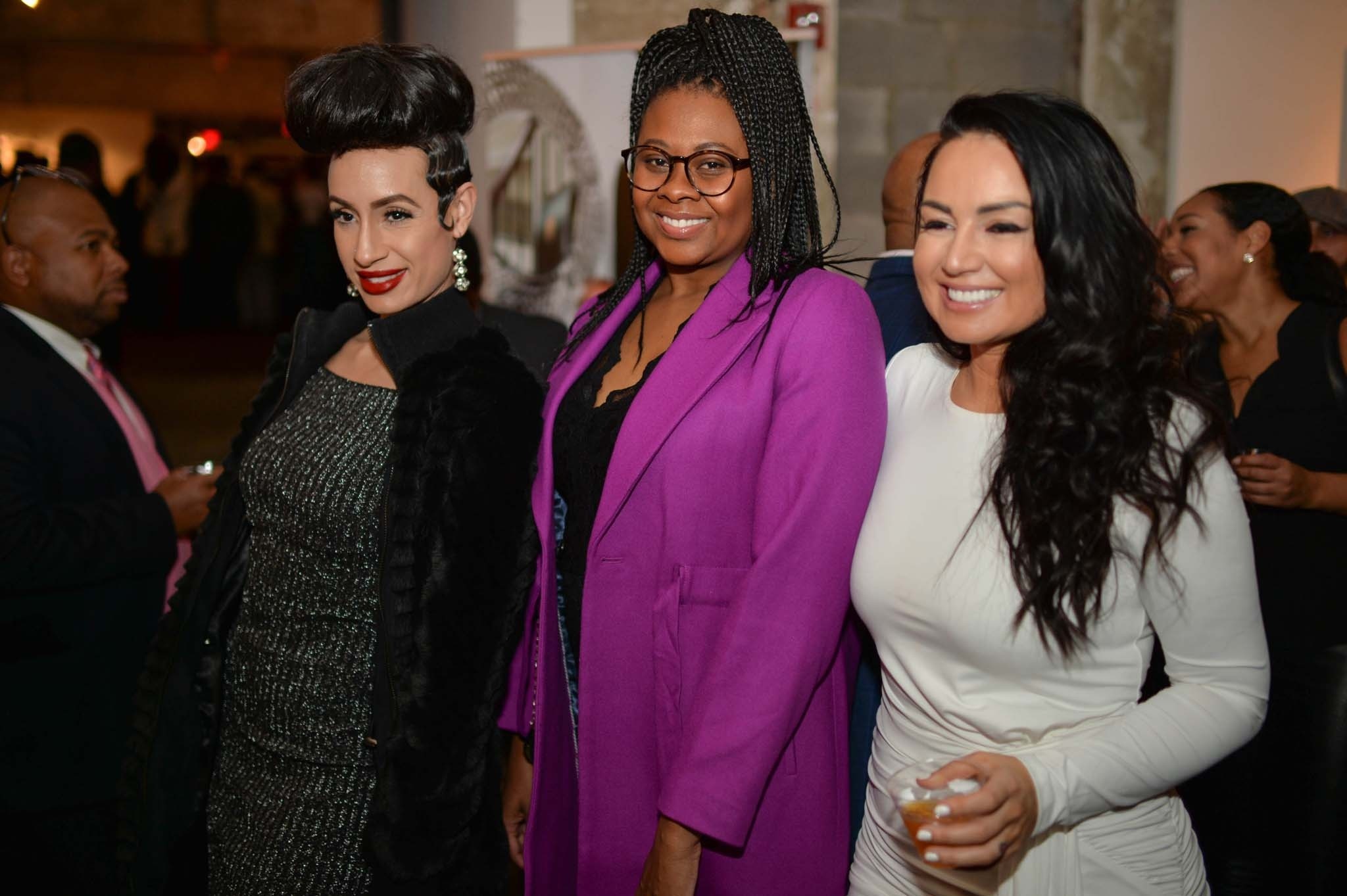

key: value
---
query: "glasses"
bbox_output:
[0,162,91,246]
[620,144,752,197]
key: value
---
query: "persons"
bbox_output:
[177,45,544,896]
[495,7,890,896]
[0,122,325,896]
[847,87,1347,896]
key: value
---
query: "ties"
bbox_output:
[85,344,193,616]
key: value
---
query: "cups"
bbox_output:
[885,758,990,869]
